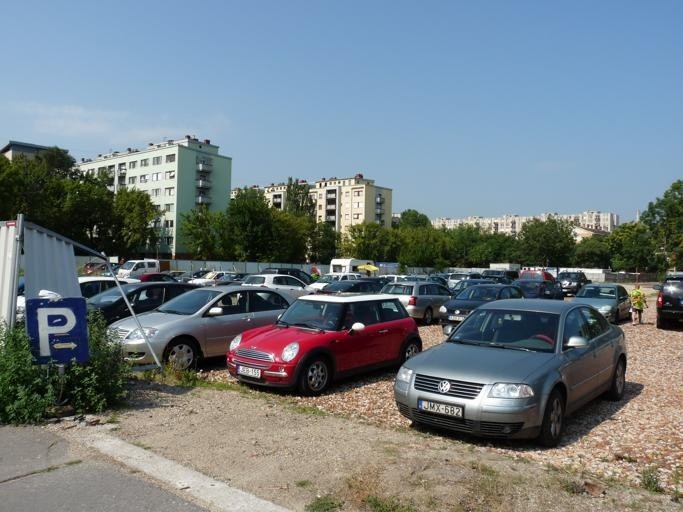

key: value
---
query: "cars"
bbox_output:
[396,298,632,449]
[107,283,298,376]
[225,291,425,397]
[570,283,632,325]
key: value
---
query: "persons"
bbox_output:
[626,283,648,325]
[310,267,320,282]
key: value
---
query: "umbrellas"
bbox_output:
[356,263,380,277]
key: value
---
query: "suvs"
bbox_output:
[651,272,682,328]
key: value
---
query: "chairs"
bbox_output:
[145,290,154,303]
[221,295,232,314]
